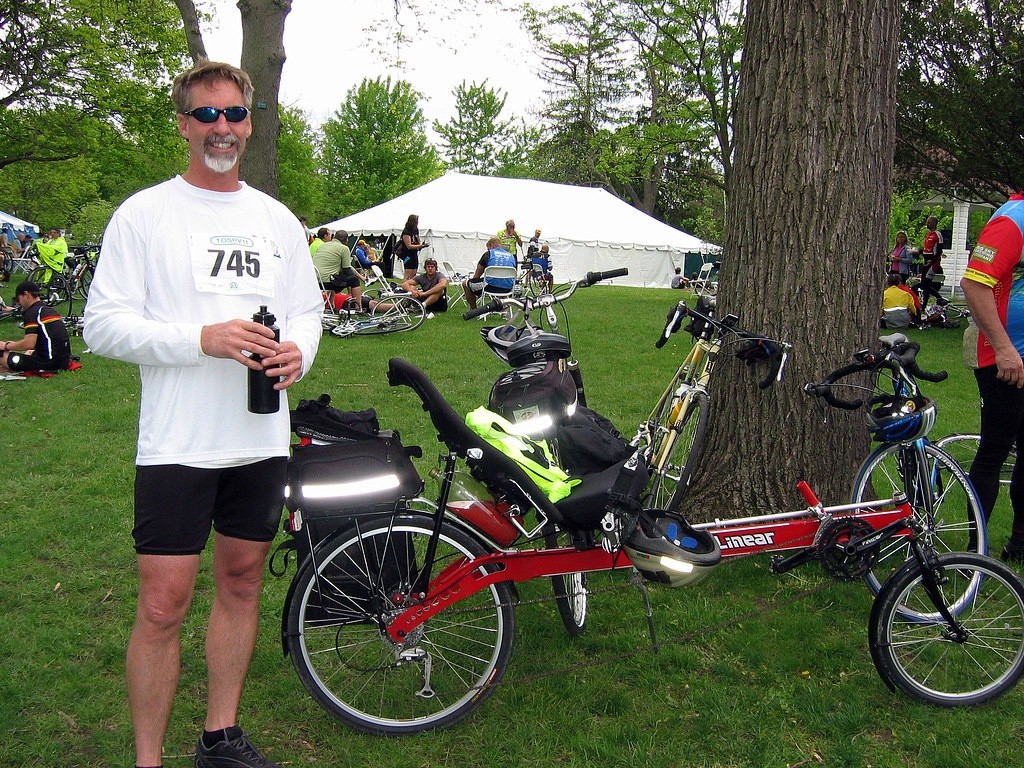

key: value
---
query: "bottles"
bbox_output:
[247,306,279,414]
[667,381,690,417]
[668,390,689,426]
[427,467,525,550]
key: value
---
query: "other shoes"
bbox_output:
[1001,539,1024,564]
[405,306,422,314]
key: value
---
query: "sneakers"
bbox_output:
[195,725,277,768]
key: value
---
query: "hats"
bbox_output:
[15,281,38,295]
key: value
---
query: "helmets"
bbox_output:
[623,510,721,588]
[863,394,939,443]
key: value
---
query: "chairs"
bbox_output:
[916,273,945,309]
[690,262,714,299]
[11,245,34,275]
[312,261,550,324]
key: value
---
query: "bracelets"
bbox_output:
[5,342,11,351]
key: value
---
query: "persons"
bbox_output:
[883,216,948,330]
[0,226,68,311]
[959,189,1024,579]
[462,219,553,320]
[321,291,402,312]
[0,281,70,371]
[298,215,379,313]
[671,267,710,289]
[401,258,448,312]
[84,63,323,768]
[401,215,431,281]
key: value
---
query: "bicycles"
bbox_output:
[322,291,426,337]
[631,294,791,512]
[462,265,631,636]
[0,242,102,336]
[935,432,1019,485]
[803,333,988,623]
[516,252,547,297]
[279,356,1024,736]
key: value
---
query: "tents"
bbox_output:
[0,211,39,238]
[310,173,722,288]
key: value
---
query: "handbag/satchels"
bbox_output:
[557,404,644,476]
[392,239,409,260]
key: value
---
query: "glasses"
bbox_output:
[185,105,252,123]
